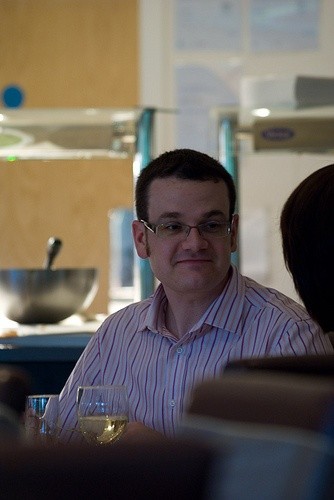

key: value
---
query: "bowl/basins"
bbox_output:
[0,268,98,327]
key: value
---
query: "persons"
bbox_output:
[46,150,334,448]
[279,164,334,332]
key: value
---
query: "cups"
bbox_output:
[75,384,128,446]
[27,394,59,442]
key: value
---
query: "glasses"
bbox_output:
[140,219,233,243]
